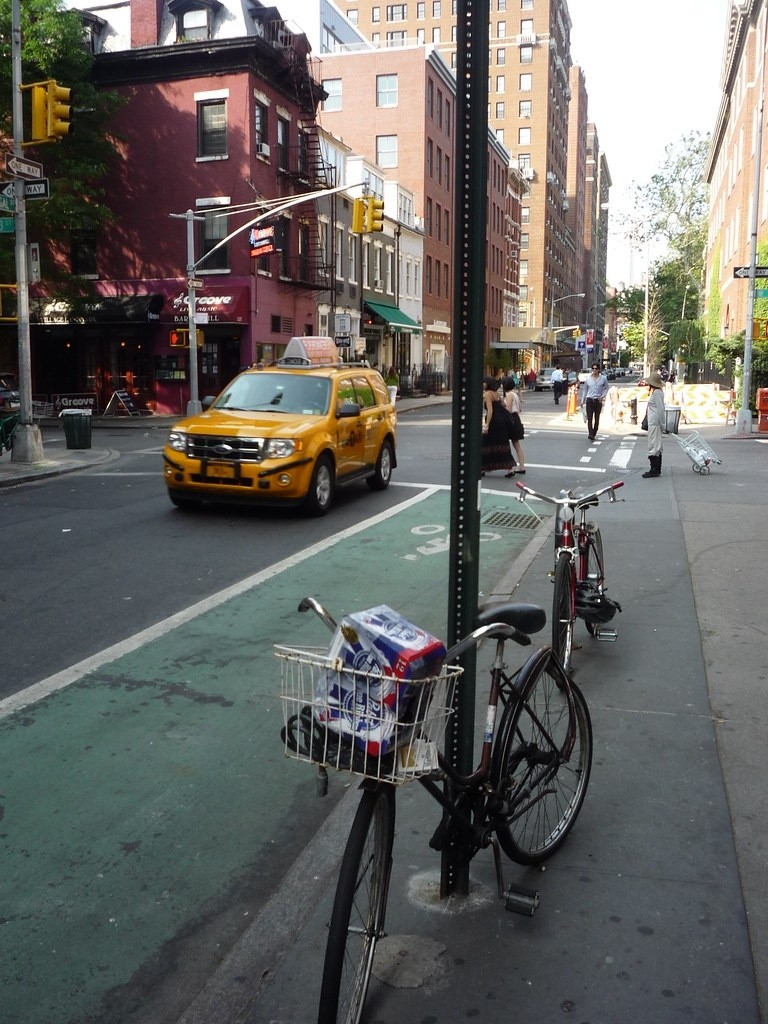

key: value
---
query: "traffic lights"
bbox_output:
[367,197,384,233]
[47,81,73,140]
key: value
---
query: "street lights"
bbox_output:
[550,285,586,332]
[586,303,607,329]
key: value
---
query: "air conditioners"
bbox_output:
[511,168,569,284]
[375,279,381,288]
[256,143,270,157]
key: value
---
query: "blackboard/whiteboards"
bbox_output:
[105,390,139,414]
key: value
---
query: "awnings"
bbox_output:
[363,299,423,335]
[0,286,251,327]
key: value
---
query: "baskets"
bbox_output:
[271,644,463,787]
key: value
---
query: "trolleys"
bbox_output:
[664,428,722,476]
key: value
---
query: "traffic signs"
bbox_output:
[5,152,43,180]
[733,266,768,279]
[0,177,50,201]
[187,277,204,290]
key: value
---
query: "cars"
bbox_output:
[162,335,398,519]
[535,368,632,392]
[0,372,21,421]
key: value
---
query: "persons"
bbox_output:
[372,362,380,372]
[385,366,400,406]
[497,364,578,405]
[480,376,515,479]
[581,363,609,440]
[499,377,526,474]
[642,372,667,478]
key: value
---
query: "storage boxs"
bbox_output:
[312,603,447,757]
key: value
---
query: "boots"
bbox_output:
[642,455,662,478]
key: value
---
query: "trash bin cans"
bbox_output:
[61,409,92,449]
[662,407,680,435]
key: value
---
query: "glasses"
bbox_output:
[592,368,598,370]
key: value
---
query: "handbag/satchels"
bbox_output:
[641,416,648,431]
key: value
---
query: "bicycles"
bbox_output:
[514,479,627,689]
[271,594,596,1024]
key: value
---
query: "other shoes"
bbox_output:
[588,433,595,440]
[514,469,525,474]
[505,472,515,478]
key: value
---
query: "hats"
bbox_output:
[645,372,667,388]
